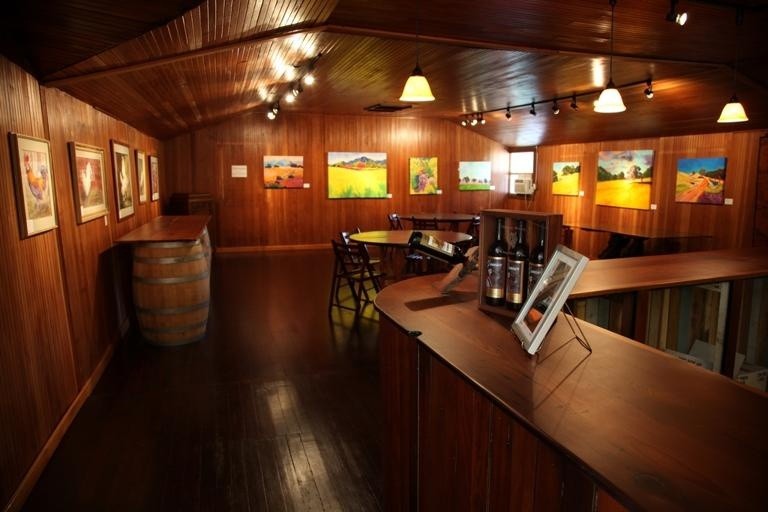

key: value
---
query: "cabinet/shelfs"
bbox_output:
[479,208,563,321]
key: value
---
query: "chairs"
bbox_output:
[327,227,379,325]
[388,211,403,230]
[412,215,437,230]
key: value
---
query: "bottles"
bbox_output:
[486,219,506,304]
[506,220,526,312]
[527,221,545,308]
[407,230,467,265]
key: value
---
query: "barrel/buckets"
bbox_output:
[196,227,214,270]
[130,239,213,348]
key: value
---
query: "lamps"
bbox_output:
[593,0,626,113]
[460,96,580,126]
[663,2,688,26]
[643,79,654,98]
[399,0,435,102]
[266,56,319,121]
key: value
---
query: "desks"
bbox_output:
[400,212,477,230]
[173,189,216,214]
[113,213,213,349]
[566,225,716,257]
[348,230,473,280]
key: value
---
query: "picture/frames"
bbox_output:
[135,149,147,205]
[110,138,135,222]
[6,130,58,240]
[148,155,160,202]
[510,245,589,356]
[67,143,110,226]
